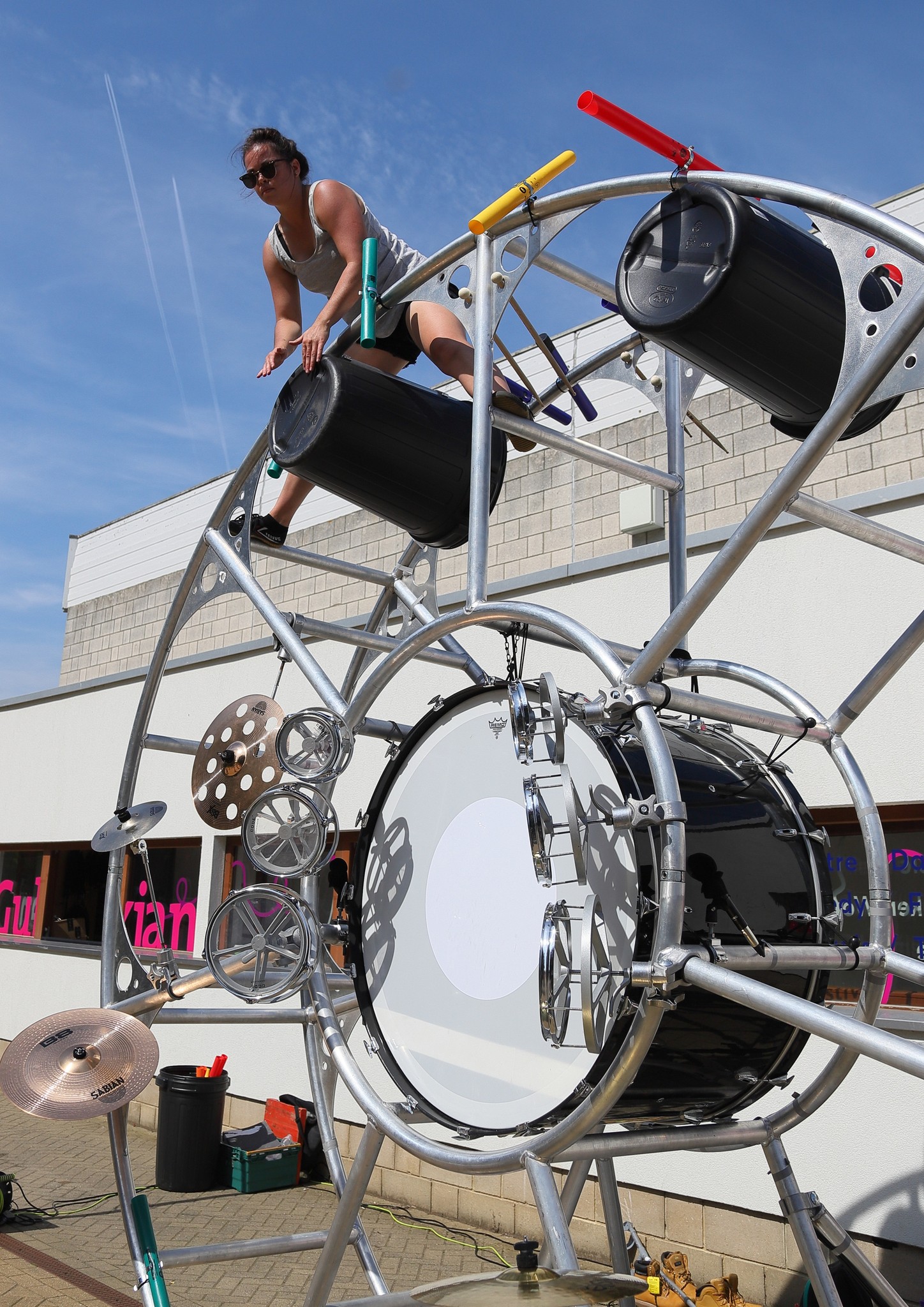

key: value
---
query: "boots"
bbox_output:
[634,1250,697,1307]
[695,1272,762,1307]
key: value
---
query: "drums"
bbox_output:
[202,883,323,1001]
[506,673,564,767]
[523,762,588,889]
[242,781,341,879]
[343,681,833,1137]
[275,705,356,786]
[538,893,608,1054]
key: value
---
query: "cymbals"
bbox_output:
[191,694,289,830]
[1,1008,160,1121]
[91,801,167,852]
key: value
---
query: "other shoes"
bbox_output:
[229,513,287,548]
[493,391,538,452]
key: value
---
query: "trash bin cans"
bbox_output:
[155,1066,231,1193]
[614,182,905,441]
[265,354,508,551]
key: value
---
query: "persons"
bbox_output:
[227,126,537,550]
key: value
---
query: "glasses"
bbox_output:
[239,158,285,189]
